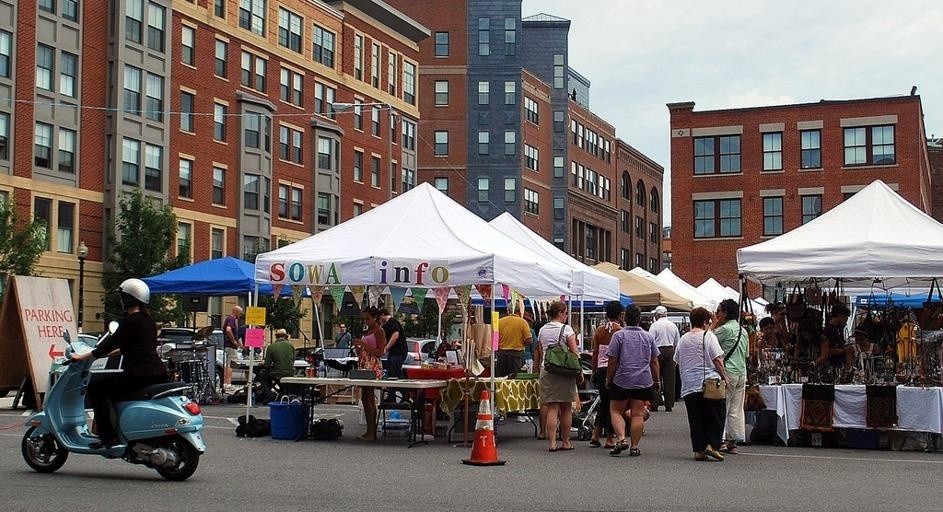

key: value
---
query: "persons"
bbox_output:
[709,299,750,454]
[351,306,387,442]
[498,300,533,423]
[335,322,352,348]
[259,328,295,401]
[534,324,563,441]
[590,301,626,449]
[537,301,584,452]
[674,307,729,461]
[604,304,661,457]
[518,311,537,373]
[377,308,409,403]
[757,300,879,372]
[69,278,168,448]
[649,305,680,412]
[221,305,244,394]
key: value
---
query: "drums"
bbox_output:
[196,348,209,361]
[182,363,203,386]
[171,348,197,363]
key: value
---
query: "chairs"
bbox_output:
[374,389,425,442]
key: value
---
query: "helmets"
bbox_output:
[113,278,151,304]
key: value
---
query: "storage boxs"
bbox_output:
[320,384,362,404]
[745,410,777,437]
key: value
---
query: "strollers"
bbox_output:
[559,353,618,441]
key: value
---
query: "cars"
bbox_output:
[405,338,441,365]
[76,333,98,349]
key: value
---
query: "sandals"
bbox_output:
[724,444,738,454]
[719,442,727,451]
[549,438,641,457]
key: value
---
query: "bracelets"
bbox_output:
[655,382,661,384]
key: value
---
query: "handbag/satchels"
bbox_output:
[742,385,765,410]
[703,379,726,400]
[544,344,582,380]
[740,312,757,359]
[787,286,942,332]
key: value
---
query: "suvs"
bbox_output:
[156,327,258,393]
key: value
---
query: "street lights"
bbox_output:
[332,102,392,200]
[77,241,87,334]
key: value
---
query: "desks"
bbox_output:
[776,383,942,447]
[439,377,540,443]
[280,377,448,448]
[401,366,490,435]
[745,384,784,448]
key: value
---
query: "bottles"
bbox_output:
[318,357,325,378]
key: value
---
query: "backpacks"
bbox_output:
[310,418,343,442]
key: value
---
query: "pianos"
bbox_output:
[230,359,311,369]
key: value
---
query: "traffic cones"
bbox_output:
[461,390,506,465]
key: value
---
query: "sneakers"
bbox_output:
[516,415,532,423]
[88,438,122,449]
[695,452,708,461]
[705,444,724,460]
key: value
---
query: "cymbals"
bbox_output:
[195,326,215,341]
[200,343,219,349]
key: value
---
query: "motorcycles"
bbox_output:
[21,321,206,481]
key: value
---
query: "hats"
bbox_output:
[275,328,290,338]
[651,306,667,314]
